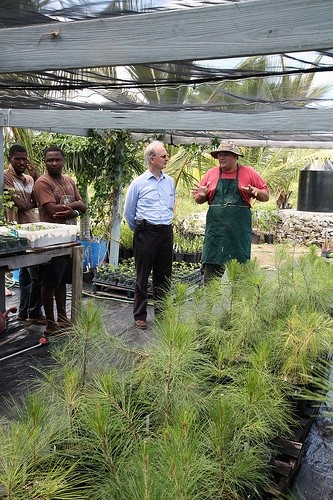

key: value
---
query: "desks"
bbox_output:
[0,243,84,328]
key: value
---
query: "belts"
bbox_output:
[149,225,172,228]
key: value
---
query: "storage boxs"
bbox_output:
[0,221,80,248]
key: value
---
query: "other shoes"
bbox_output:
[16,316,28,321]
[136,319,148,330]
[26,316,46,325]
[44,328,66,337]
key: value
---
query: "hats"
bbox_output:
[211,140,244,159]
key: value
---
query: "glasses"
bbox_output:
[156,153,169,158]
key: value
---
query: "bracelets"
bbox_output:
[74,210,79,215]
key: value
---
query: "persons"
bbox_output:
[31,148,86,336]
[4,144,46,321]
[124,141,176,329]
[191,140,270,286]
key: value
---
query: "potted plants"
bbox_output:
[94,229,205,298]
[250,207,281,244]
